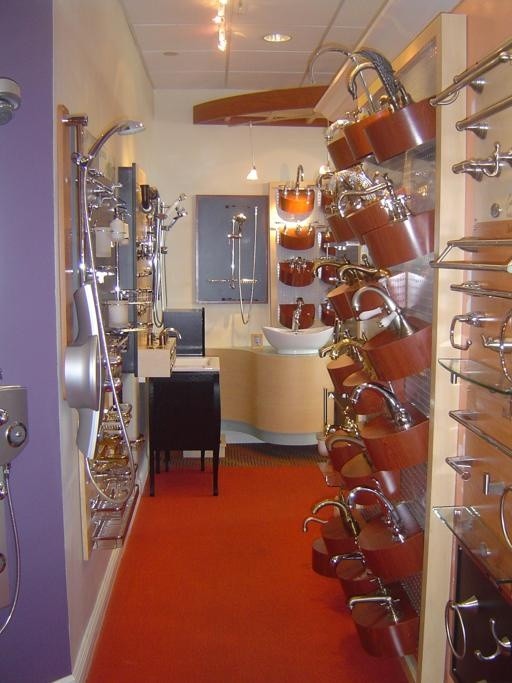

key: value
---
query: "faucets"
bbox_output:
[293,43,417,620]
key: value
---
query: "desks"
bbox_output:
[145,356,221,497]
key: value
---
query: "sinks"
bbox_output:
[264,323,335,354]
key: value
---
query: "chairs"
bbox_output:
[163,306,206,357]
